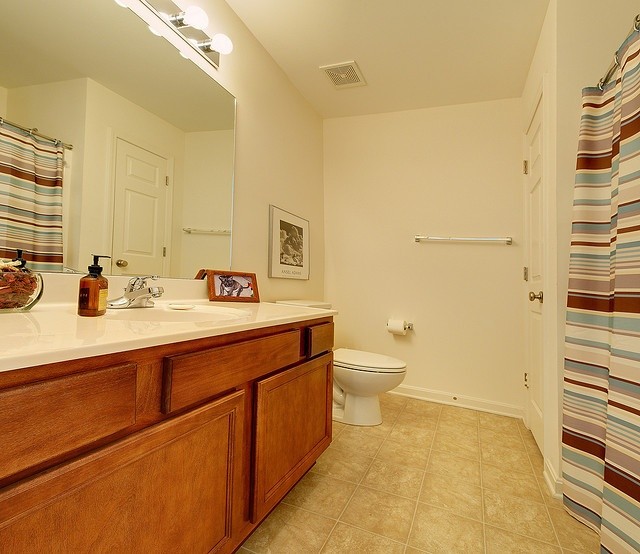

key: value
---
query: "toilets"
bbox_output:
[276,300,407,426]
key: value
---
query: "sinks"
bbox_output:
[103,304,249,323]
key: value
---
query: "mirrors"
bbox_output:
[0,0,235,276]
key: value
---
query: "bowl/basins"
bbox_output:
[0,270,44,313]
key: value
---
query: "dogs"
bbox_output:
[218,275,251,297]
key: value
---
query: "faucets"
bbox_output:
[107,275,164,309]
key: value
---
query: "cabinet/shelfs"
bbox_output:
[1,299,335,554]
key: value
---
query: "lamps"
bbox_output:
[170,4,209,32]
[195,32,234,56]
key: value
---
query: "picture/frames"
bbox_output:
[269,204,310,279]
[206,269,259,300]
